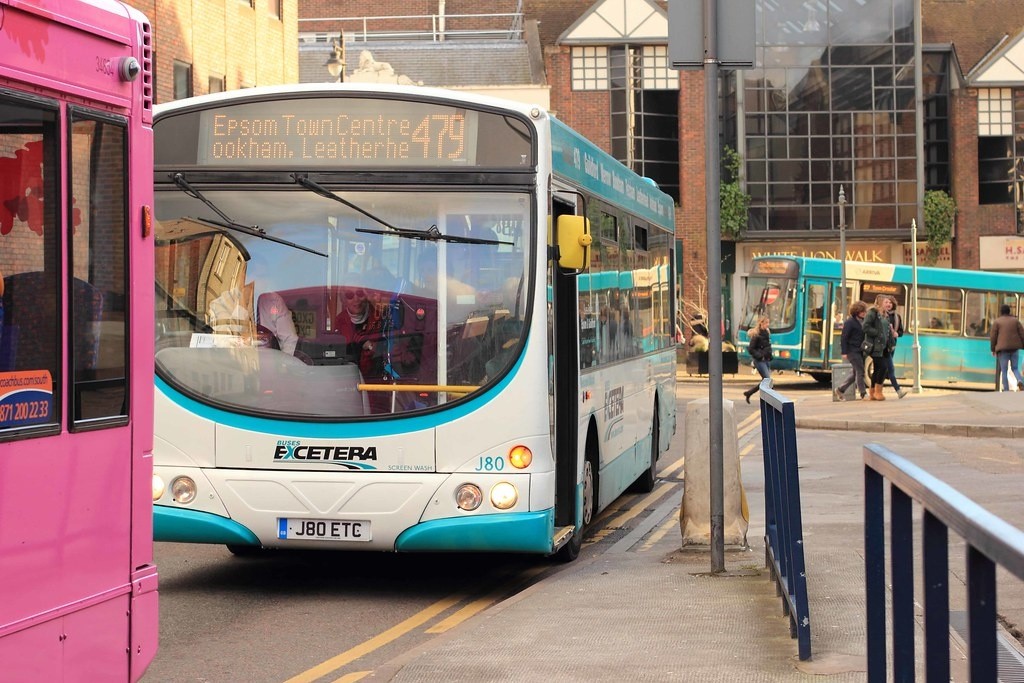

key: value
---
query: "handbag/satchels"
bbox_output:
[859,341,873,355]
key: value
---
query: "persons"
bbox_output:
[584,286,639,359]
[744,291,994,338]
[990,305,1023,391]
[886,298,908,400]
[209,252,298,360]
[330,274,385,379]
[743,314,773,404]
[863,295,890,401]
[835,301,870,400]
[382,226,523,374]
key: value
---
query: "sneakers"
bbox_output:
[862,394,870,400]
[835,388,846,402]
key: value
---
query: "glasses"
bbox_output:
[344,290,363,299]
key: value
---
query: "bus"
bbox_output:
[736,255,1024,391]
[0,0,159,683]
[152,81,683,567]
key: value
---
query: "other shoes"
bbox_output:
[897,390,908,399]
[1018,382,1024,391]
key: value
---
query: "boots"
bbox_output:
[870,383,885,401]
[744,388,757,404]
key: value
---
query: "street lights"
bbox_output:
[321,28,346,84]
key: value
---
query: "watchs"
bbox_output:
[366,344,373,351]
